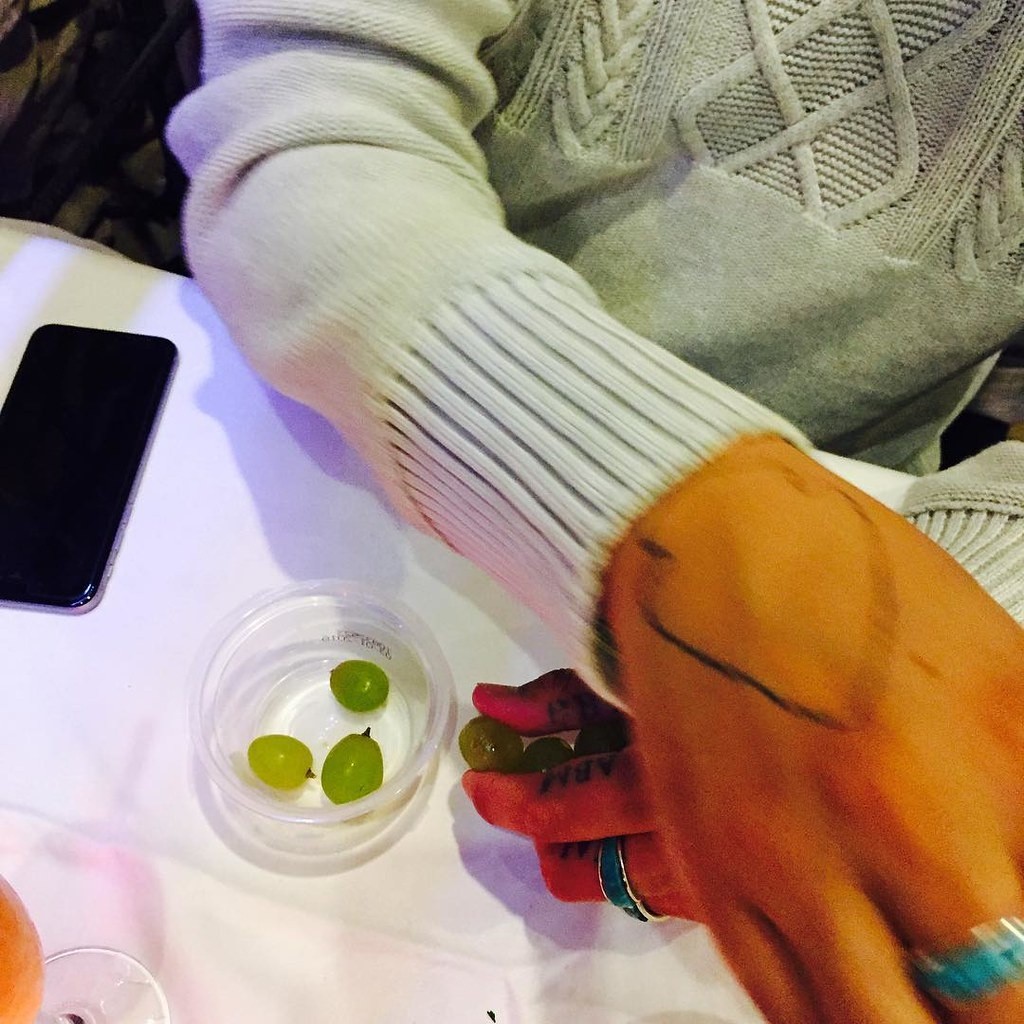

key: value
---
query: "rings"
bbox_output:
[908,914,1024,1002]
[597,834,673,924]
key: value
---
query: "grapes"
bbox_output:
[247,661,390,806]
[458,714,626,775]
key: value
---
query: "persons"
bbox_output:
[161,2,1023,1023]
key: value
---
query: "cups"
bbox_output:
[184,584,451,829]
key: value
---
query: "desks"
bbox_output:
[3,216,922,1024]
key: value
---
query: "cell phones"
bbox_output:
[0,323,179,615]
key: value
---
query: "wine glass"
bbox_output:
[0,875,173,1024]
